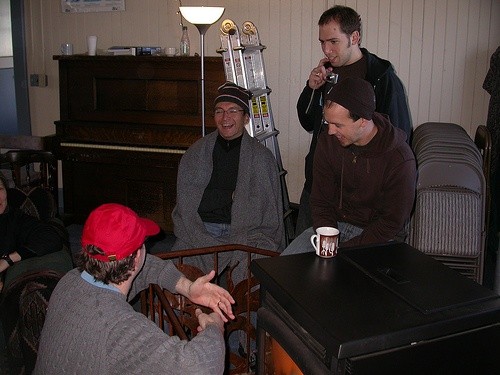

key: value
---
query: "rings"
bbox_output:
[317,73,320,77]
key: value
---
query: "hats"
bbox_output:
[80,204,161,262]
[215,81,253,113]
[327,76,377,119]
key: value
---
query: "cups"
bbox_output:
[310,227,339,258]
[87,35,97,55]
[60,43,73,55]
[164,47,179,57]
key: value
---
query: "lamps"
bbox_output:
[179,6,226,137]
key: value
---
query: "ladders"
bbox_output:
[216,18,297,248]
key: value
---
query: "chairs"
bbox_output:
[0,135,74,375]
[404,123,493,285]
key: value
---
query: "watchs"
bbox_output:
[0,253,14,267]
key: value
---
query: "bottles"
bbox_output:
[180,26,191,56]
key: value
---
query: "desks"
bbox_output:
[250,242,500,375]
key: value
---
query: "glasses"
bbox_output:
[305,72,338,114]
[214,106,245,114]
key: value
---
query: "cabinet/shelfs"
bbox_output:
[52,56,228,238]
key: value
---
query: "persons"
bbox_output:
[0,174,69,291]
[148,81,286,375]
[33,203,235,375]
[277,5,419,257]
